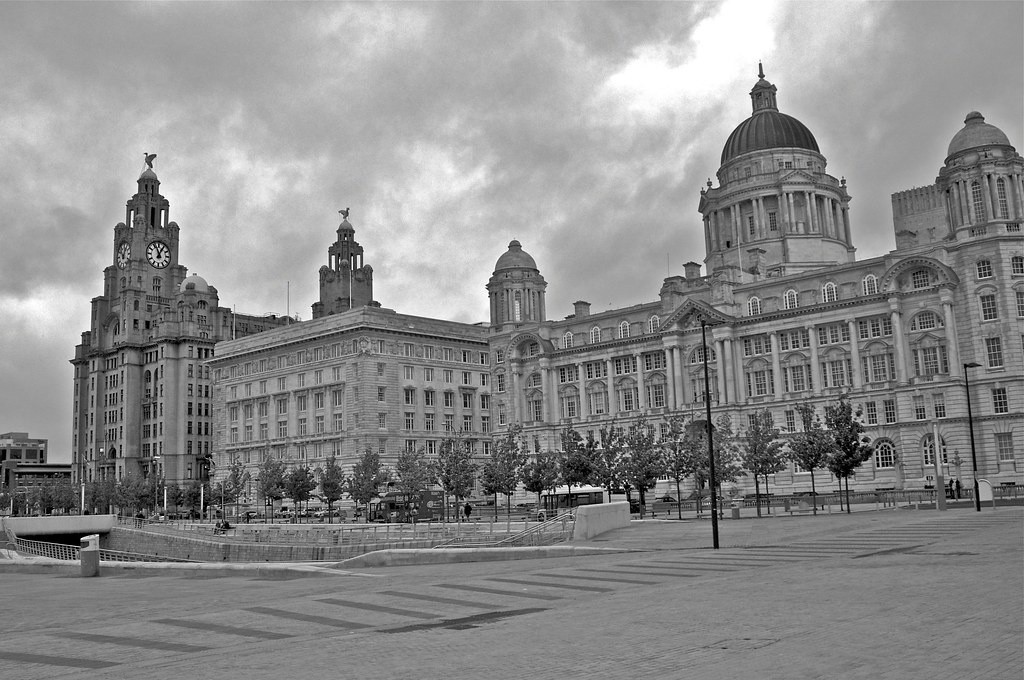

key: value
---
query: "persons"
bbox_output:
[949,478,955,499]
[464,502,472,521]
[955,478,961,499]
[214,520,229,535]
[460,505,465,522]
[134,511,144,529]
[84,507,89,515]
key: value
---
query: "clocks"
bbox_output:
[116,241,132,271]
[147,241,172,270]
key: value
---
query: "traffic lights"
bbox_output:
[204,454,211,470]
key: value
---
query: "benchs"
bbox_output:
[341,519,357,524]
[786,507,818,515]
[249,520,266,524]
[310,519,324,523]
[651,502,671,514]
[792,497,826,511]
[277,519,288,524]
[698,512,724,520]
[213,527,238,535]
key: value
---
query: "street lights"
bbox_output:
[962,362,983,512]
[697,313,721,550]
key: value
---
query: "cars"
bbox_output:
[655,496,679,503]
[612,485,625,495]
[629,499,641,514]
[237,503,368,518]
[701,495,725,502]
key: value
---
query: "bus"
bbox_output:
[539,488,608,521]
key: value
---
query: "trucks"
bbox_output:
[368,489,445,523]
